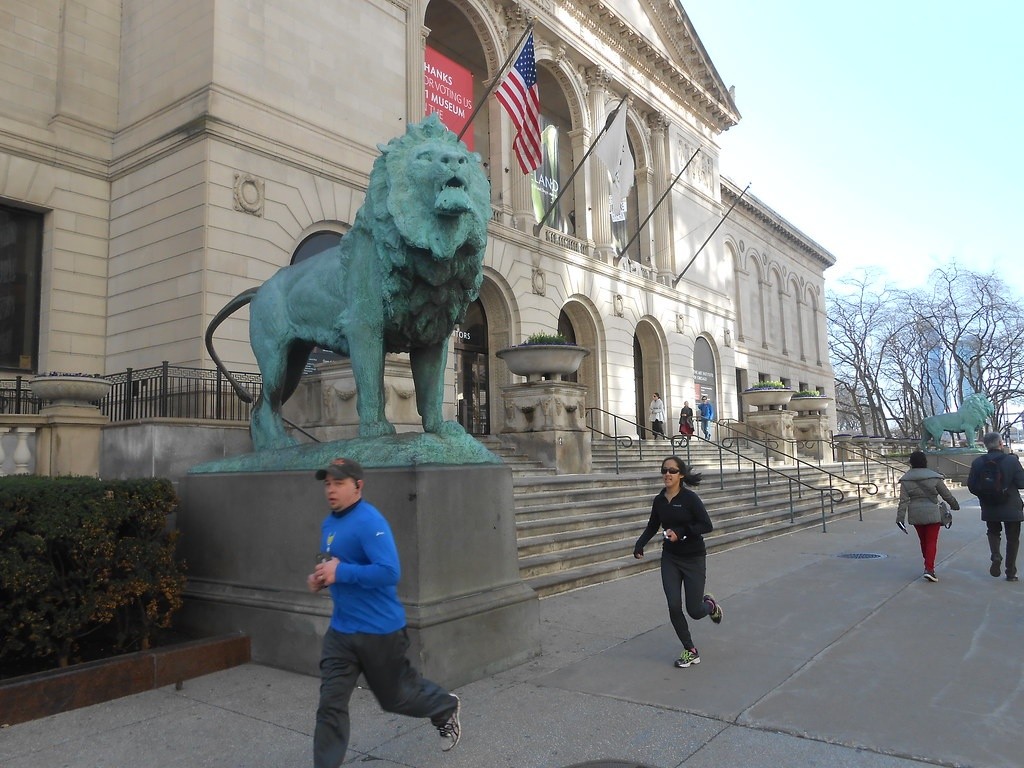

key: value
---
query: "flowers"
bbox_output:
[833,434,884,438]
[791,389,827,398]
[494,330,576,346]
[745,380,792,389]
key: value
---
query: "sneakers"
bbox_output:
[674,648,700,668]
[436,694,461,752]
[704,594,722,624]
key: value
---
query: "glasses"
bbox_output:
[660,468,679,474]
[654,396,657,397]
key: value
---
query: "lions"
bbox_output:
[205,111,491,453]
[904,393,994,452]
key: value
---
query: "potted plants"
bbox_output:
[27,372,114,405]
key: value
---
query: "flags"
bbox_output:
[493,28,543,175]
[593,101,634,216]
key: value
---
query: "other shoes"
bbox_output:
[1006,575,1018,581]
[923,571,938,582]
[708,434,711,440]
[990,558,1001,577]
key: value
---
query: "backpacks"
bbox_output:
[979,454,1009,501]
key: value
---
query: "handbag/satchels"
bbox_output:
[938,502,952,529]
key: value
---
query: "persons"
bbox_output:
[679,401,694,439]
[649,393,665,440]
[896,453,960,582]
[633,456,723,667]
[306,458,460,768]
[968,433,1024,581]
[698,396,714,441]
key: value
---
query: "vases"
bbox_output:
[789,397,833,415]
[741,390,795,410]
[834,436,894,443]
[495,345,592,381]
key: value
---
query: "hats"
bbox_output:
[315,457,364,481]
